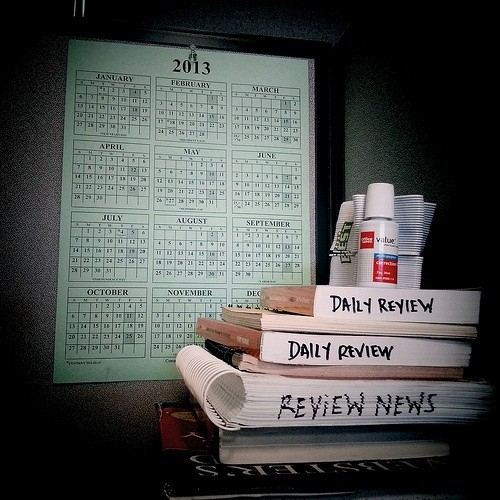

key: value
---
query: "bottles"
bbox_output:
[357,181,399,290]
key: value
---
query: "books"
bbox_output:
[220,306,478,341]
[261,283,482,326]
[194,316,472,368]
[186,391,450,466]
[203,339,465,380]
[153,401,211,454]
[175,345,495,433]
